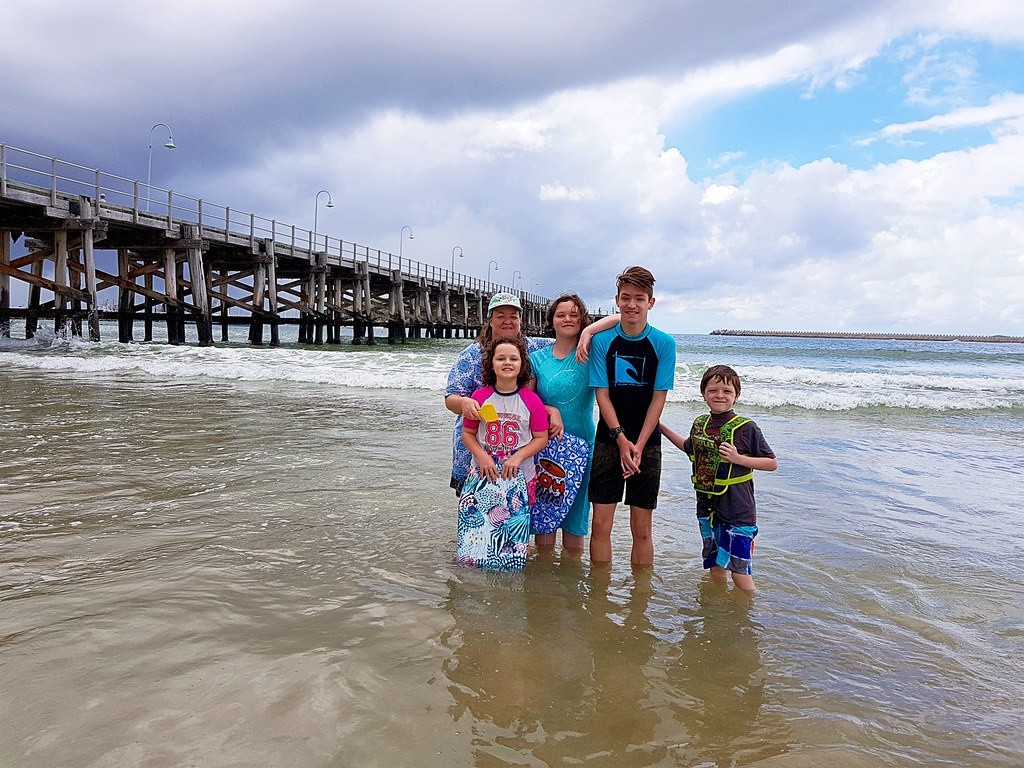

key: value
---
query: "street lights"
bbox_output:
[451,246,464,284]
[314,190,335,251]
[487,260,498,293]
[399,226,415,272]
[512,270,522,290]
[146,123,176,213]
[531,277,538,293]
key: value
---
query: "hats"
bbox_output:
[488,293,523,318]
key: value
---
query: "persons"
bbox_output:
[444,292,624,573]
[658,363,778,592]
[588,265,677,566]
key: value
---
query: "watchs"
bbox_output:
[608,426,626,440]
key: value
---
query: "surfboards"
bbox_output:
[455,452,532,575]
